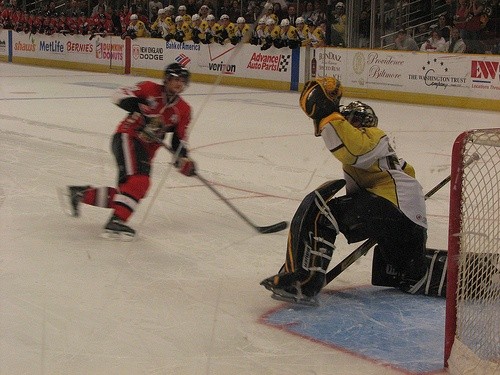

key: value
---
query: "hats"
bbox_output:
[398,28,406,34]
[307,21,317,27]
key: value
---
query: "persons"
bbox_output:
[0,0,500,54]
[259,77,500,308]
[56,62,196,242]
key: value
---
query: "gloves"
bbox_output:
[176,156,197,178]
[120,30,136,40]
[135,117,162,140]
[164,31,185,43]
[151,30,163,39]
[192,28,242,46]
[249,35,302,51]
[2,23,107,39]
[299,77,345,136]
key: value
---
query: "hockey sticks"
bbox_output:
[322,152,480,288]
[144,131,290,234]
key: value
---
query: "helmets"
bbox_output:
[258,3,276,26]
[130,14,138,20]
[158,5,215,23]
[220,14,230,20]
[279,18,290,27]
[295,17,304,24]
[336,2,344,9]
[342,101,376,127]
[5,2,106,19]
[236,17,246,24]
[165,64,190,80]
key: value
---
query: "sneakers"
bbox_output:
[59,183,87,217]
[100,215,136,241]
[264,271,325,307]
